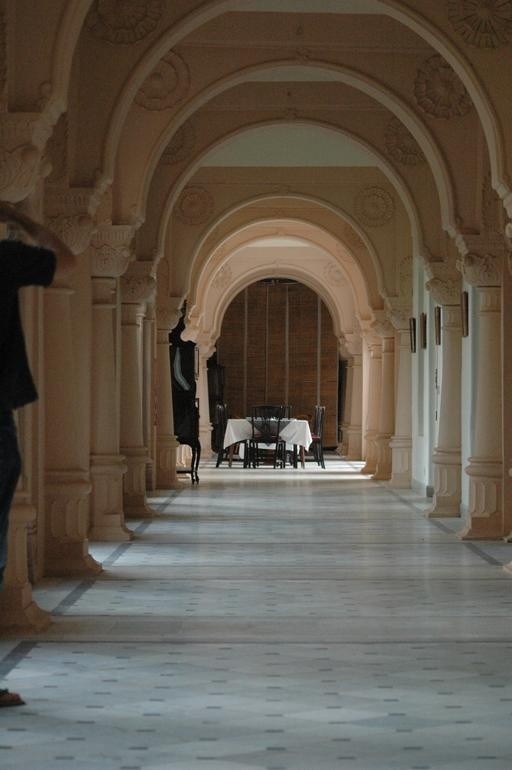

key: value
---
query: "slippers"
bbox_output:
[0,688,25,707]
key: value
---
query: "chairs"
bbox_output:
[210,401,334,469]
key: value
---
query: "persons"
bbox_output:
[0,236,57,708]
